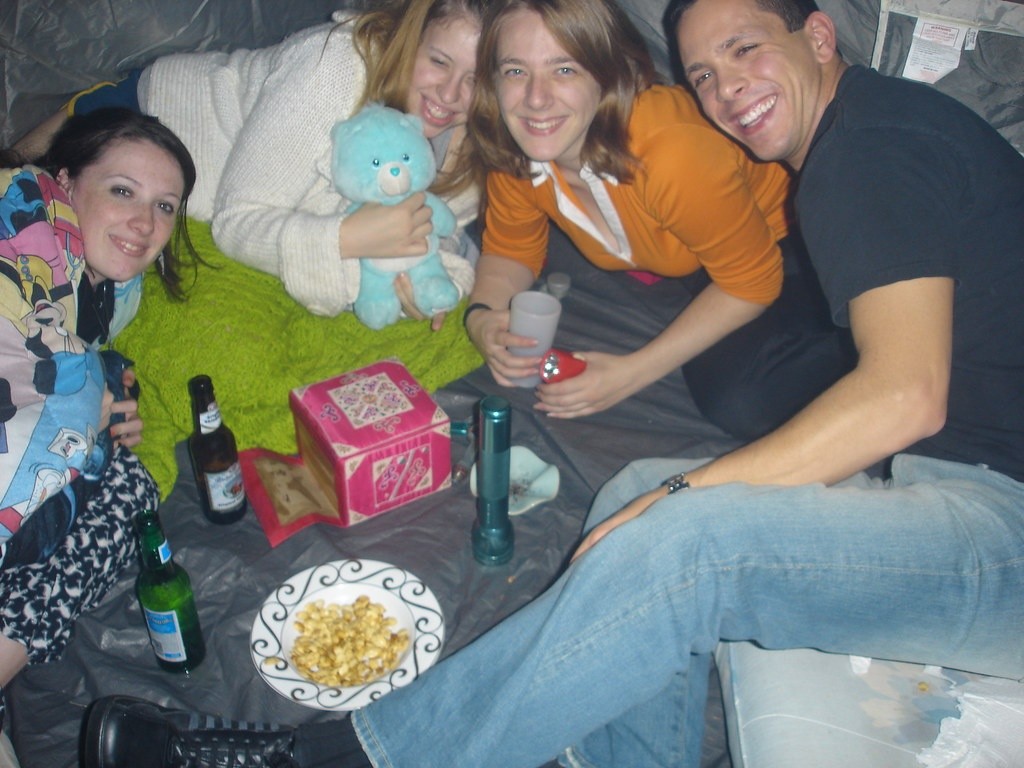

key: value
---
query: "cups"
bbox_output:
[504,290,561,389]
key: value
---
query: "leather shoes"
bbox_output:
[84,694,299,768]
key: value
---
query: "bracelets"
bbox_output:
[463,302,494,328]
[660,473,690,496]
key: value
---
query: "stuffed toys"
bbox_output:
[330,104,461,330]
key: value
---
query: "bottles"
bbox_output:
[186,373,247,524]
[133,509,205,674]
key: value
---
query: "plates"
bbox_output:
[250,557,446,713]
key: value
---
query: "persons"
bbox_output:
[462,0,859,441]
[0,106,219,768]
[78,0,1024,768]
[0,0,488,332]
